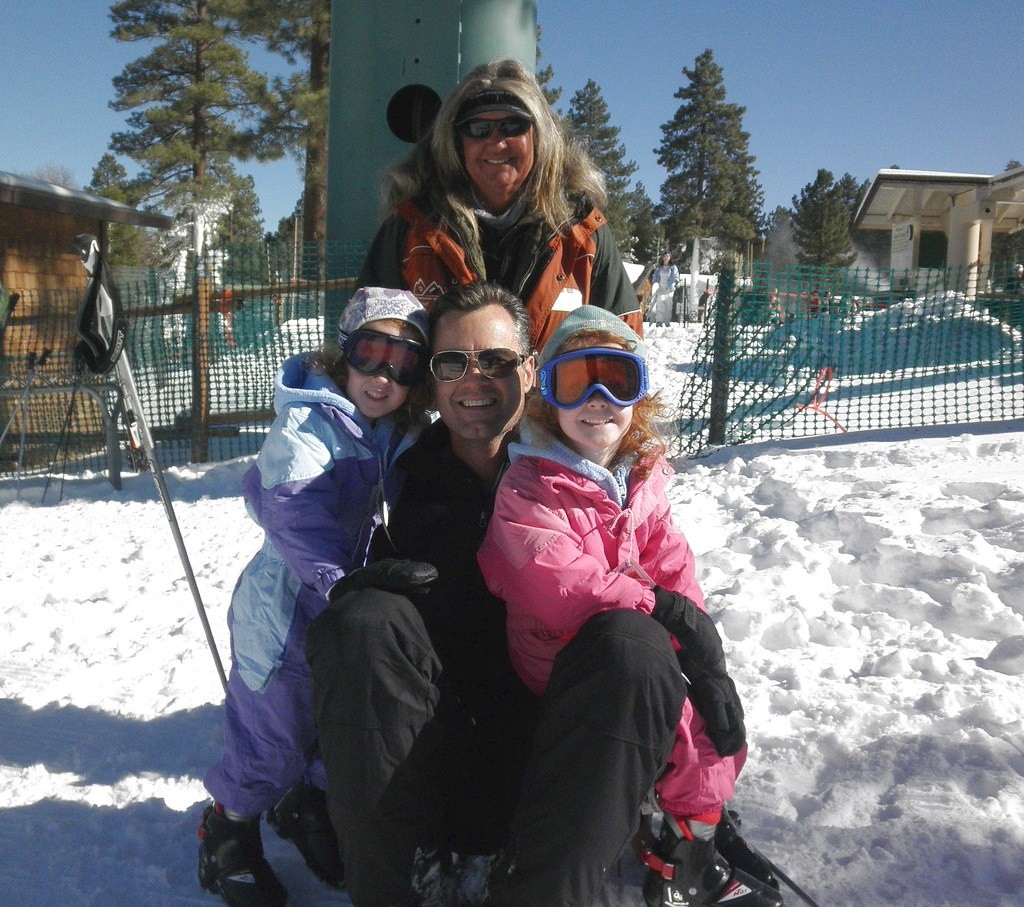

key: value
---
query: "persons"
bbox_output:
[198,285,432,907]
[354,59,645,397]
[476,303,782,907]
[807,291,860,317]
[651,250,680,328]
[305,278,686,907]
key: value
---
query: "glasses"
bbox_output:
[429,347,525,382]
[336,327,428,386]
[460,116,535,138]
[539,346,650,409]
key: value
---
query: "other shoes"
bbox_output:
[642,809,784,907]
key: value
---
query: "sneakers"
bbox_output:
[267,777,346,891]
[198,800,288,907]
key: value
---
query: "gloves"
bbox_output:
[676,650,746,756]
[328,557,438,598]
[650,584,726,679]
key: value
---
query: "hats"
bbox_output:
[337,287,430,356]
[537,305,647,369]
